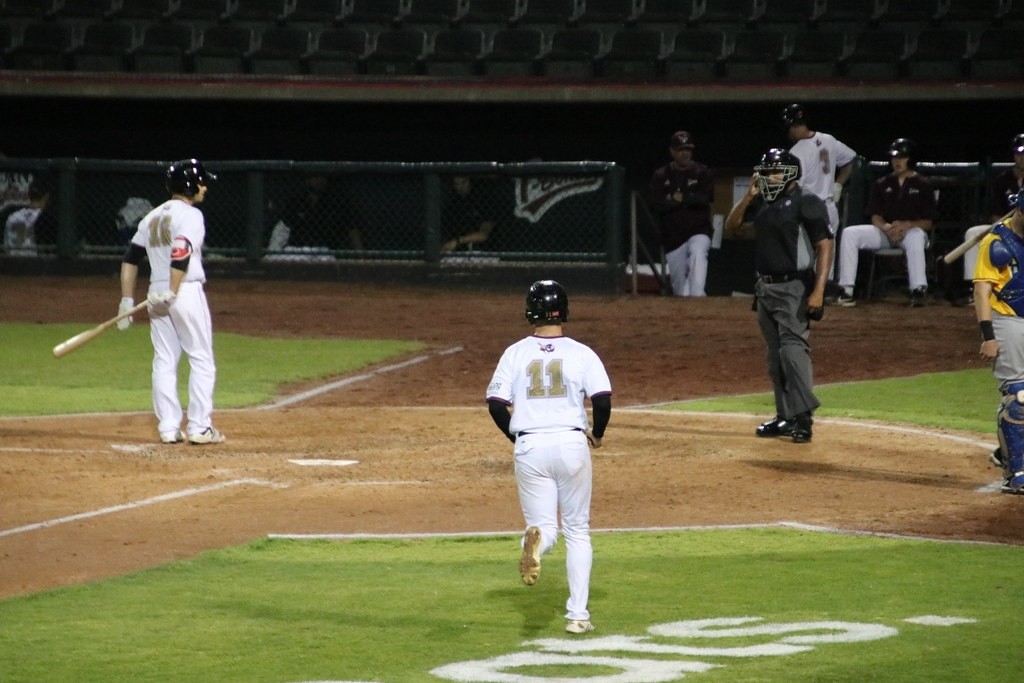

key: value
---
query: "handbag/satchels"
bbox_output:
[800,268,824,321]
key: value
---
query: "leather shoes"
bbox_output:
[756,416,796,437]
[791,411,813,442]
[518,428,581,436]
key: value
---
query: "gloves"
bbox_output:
[147,289,176,318]
[116,297,134,330]
[833,183,842,203]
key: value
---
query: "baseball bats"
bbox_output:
[943,207,1017,264]
[52,295,158,357]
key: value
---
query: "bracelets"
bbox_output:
[453,236,460,247]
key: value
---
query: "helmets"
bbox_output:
[525,279,568,325]
[166,156,219,199]
[1010,133,1024,153]
[781,103,808,129]
[888,138,918,170]
[1008,188,1024,212]
[753,148,802,202]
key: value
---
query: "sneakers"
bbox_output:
[1001,474,1024,493]
[990,447,1004,467]
[519,525,542,586]
[824,289,856,307]
[957,293,975,308]
[565,621,596,634]
[910,288,928,308]
[187,426,225,445]
[161,430,185,443]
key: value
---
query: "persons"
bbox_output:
[829,139,936,309]
[974,189,1024,494]
[723,147,833,444]
[0,170,57,271]
[116,158,228,444]
[442,175,495,252]
[990,133,1024,223]
[269,168,363,253]
[486,279,612,633]
[779,104,857,305]
[649,131,713,298]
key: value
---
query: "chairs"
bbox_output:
[0,0,1023,302]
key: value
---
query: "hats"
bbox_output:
[670,131,694,150]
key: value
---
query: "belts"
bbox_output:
[762,271,799,284]
